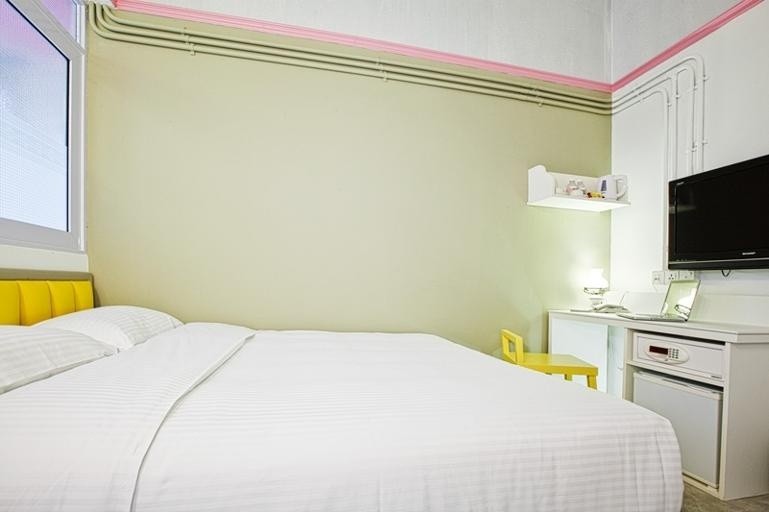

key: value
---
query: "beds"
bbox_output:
[0,268,685,512]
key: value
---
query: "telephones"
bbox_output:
[594,305,630,313]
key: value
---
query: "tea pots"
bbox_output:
[599,174,626,200]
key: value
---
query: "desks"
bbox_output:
[548,310,768,502]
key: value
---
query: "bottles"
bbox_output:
[567,179,585,195]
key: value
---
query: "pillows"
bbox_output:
[32,304,184,350]
[0,324,119,393]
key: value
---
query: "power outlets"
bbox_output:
[652,269,694,285]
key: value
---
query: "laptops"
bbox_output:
[617,280,701,322]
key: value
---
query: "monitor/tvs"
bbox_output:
[669,155,769,270]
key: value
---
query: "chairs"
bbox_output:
[500,329,598,390]
